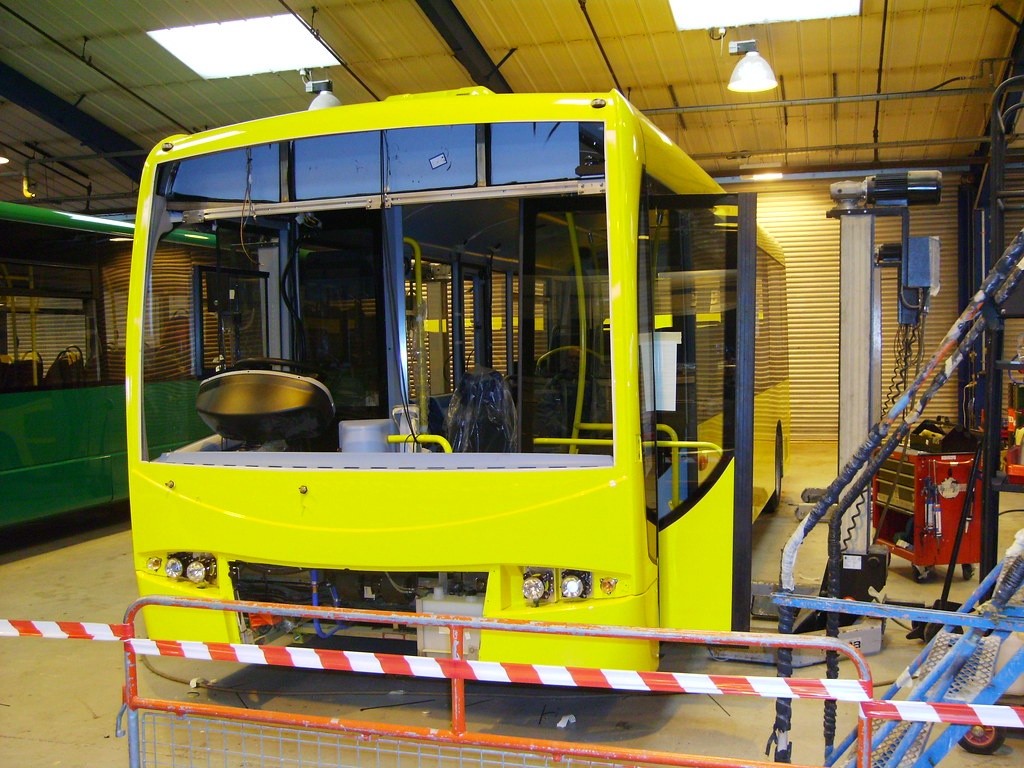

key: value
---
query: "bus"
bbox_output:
[0,201,377,529]
[124,82,789,674]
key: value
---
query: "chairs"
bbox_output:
[85,346,114,387]
[21,351,44,384]
[596,324,611,359]
[162,308,190,351]
[155,345,179,381]
[40,350,77,391]
[546,326,591,377]
[61,346,85,385]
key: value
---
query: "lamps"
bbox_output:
[299,69,343,111]
[726,40,778,93]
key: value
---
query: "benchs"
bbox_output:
[409,393,480,452]
